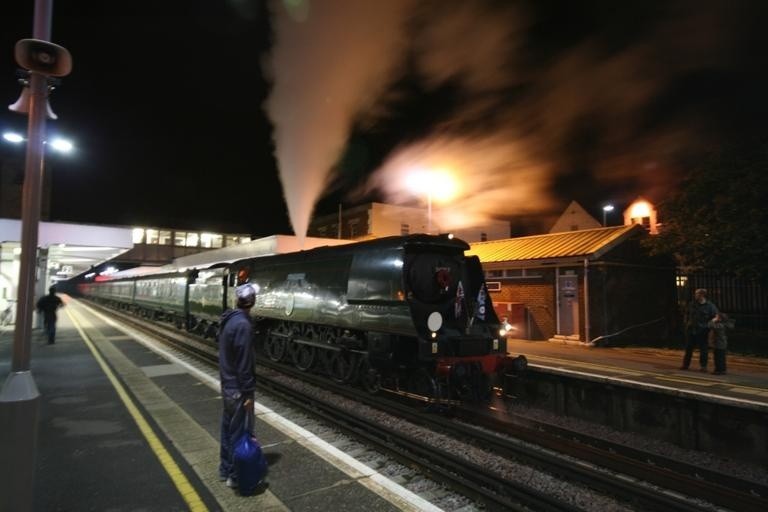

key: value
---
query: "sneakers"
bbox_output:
[219,475,238,488]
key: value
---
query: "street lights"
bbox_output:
[1,127,75,401]
[603,205,614,226]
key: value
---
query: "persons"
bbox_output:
[710,313,736,375]
[37,286,63,345]
[679,288,718,372]
[216,284,268,489]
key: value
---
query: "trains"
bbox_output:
[78,234,510,413]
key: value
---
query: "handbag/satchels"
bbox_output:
[234,432,267,497]
